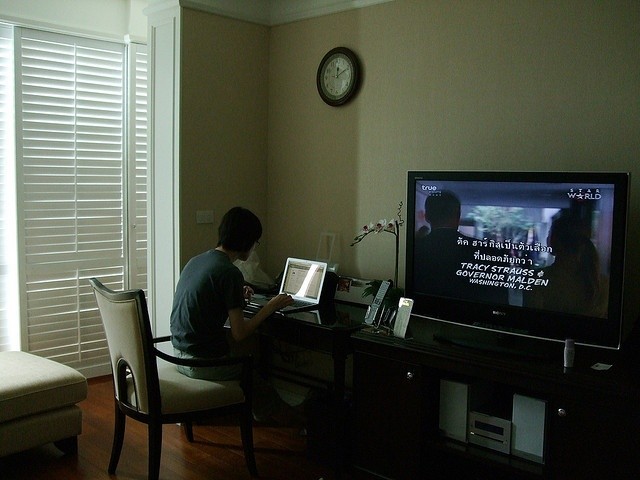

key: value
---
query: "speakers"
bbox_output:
[438,379,470,445]
[510,392,546,464]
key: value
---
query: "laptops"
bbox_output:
[245,257,328,317]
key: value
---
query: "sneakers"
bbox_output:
[250,397,273,420]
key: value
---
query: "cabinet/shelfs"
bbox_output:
[350,315,640,479]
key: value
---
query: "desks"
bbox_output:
[241,288,364,406]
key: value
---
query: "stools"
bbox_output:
[1,350,88,461]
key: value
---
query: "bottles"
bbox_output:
[564,340,576,367]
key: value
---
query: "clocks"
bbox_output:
[315,45,363,108]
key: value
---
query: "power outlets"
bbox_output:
[196,209,215,224]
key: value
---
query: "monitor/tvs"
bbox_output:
[404,170,631,359]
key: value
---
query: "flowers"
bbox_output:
[350,201,405,285]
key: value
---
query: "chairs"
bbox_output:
[88,276,263,479]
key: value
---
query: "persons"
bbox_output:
[170,206,293,422]
[418,225,429,239]
[522,208,609,320]
[414,190,494,304]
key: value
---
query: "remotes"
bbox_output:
[363,281,391,325]
